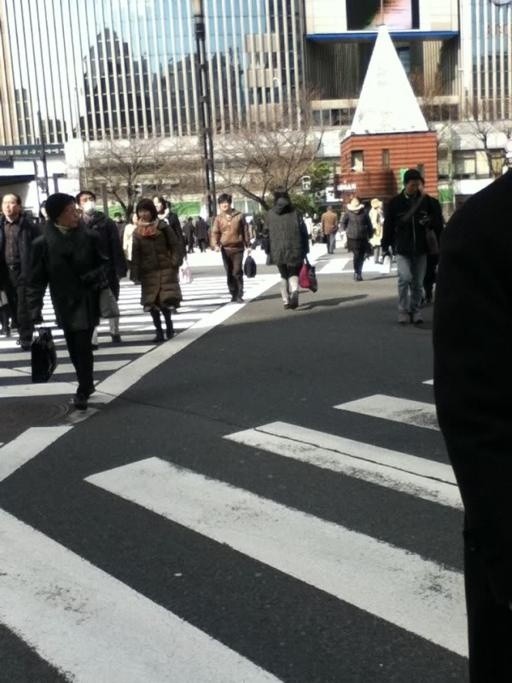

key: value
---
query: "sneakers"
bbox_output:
[412,312,423,324]
[398,312,410,324]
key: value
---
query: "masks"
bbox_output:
[80,201,96,215]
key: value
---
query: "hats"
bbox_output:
[346,198,364,211]
[45,193,75,220]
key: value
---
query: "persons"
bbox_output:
[341,194,375,282]
[381,169,444,326]
[27,193,120,410]
[433,170,511,682]
[209,194,252,304]
[261,185,310,311]
[1,189,188,350]
[182,184,394,254]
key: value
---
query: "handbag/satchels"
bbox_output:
[244,256,256,277]
[99,287,120,319]
[299,263,317,292]
[30,326,56,383]
[180,263,194,285]
